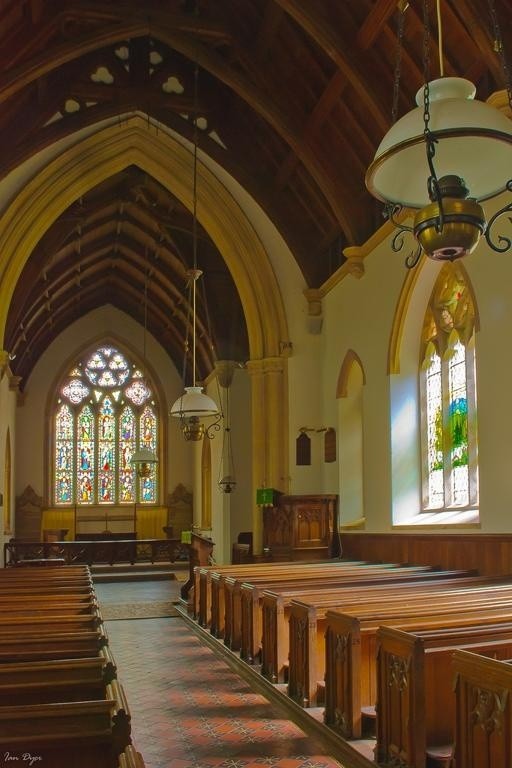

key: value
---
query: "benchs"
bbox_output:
[1,561,143,767]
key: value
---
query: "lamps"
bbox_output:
[166,2,227,443]
[364,1,510,270]
